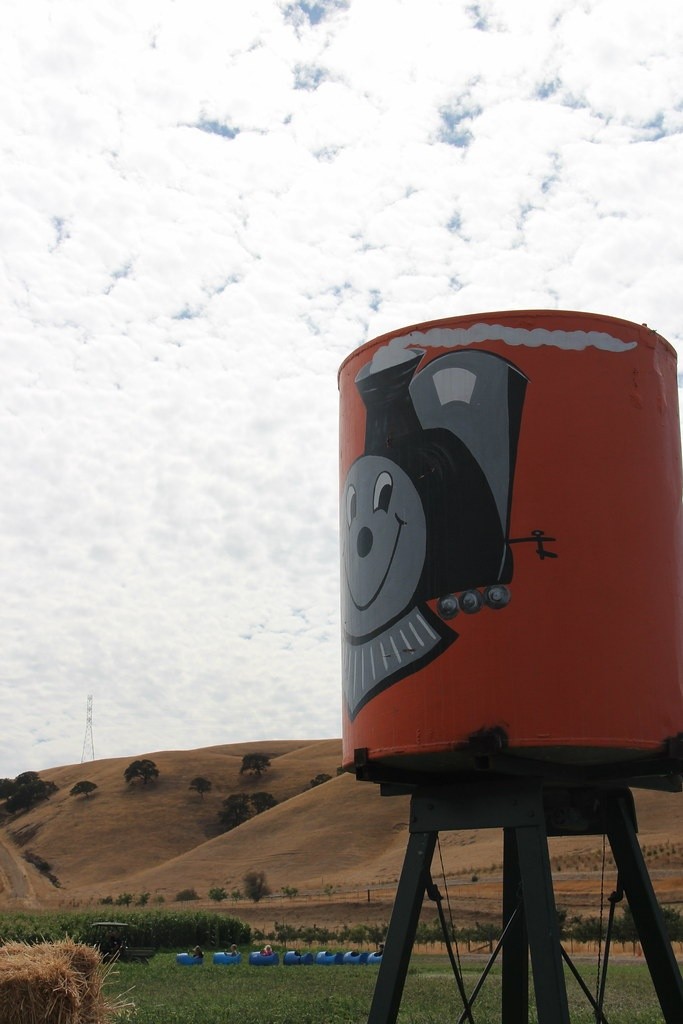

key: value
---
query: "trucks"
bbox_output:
[77,921,156,968]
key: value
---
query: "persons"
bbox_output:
[295,949,302,956]
[260,944,272,956]
[374,943,384,956]
[350,947,360,956]
[224,944,238,957]
[191,945,203,958]
